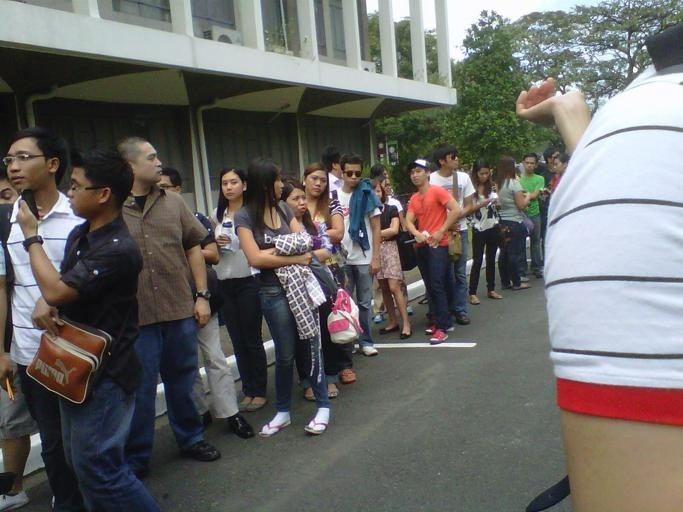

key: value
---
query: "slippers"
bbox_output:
[258,415,293,439]
[303,417,329,436]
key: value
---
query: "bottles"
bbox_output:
[219,220,232,251]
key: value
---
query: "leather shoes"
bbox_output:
[227,412,256,441]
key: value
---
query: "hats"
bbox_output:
[405,158,432,171]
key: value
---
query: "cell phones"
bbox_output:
[21,188,40,222]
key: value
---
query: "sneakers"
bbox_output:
[0,489,32,510]
[304,367,357,404]
[362,344,380,357]
[177,439,224,462]
[373,296,470,343]
[236,395,269,412]
[466,275,531,305]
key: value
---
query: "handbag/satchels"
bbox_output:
[447,229,464,262]
[392,230,419,272]
[21,316,116,409]
[521,216,536,237]
[324,288,364,347]
[496,224,513,249]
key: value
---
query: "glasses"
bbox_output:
[1,152,54,167]
[343,169,363,178]
[70,181,109,191]
[445,154,458,161]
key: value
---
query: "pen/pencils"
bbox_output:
[6,376,15,401]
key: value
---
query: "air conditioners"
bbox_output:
[202,25,242,45]
[361,60,376,72]
[272,47,293,56]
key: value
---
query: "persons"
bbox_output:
[0,127,569,512]
[516,17,682,512]
